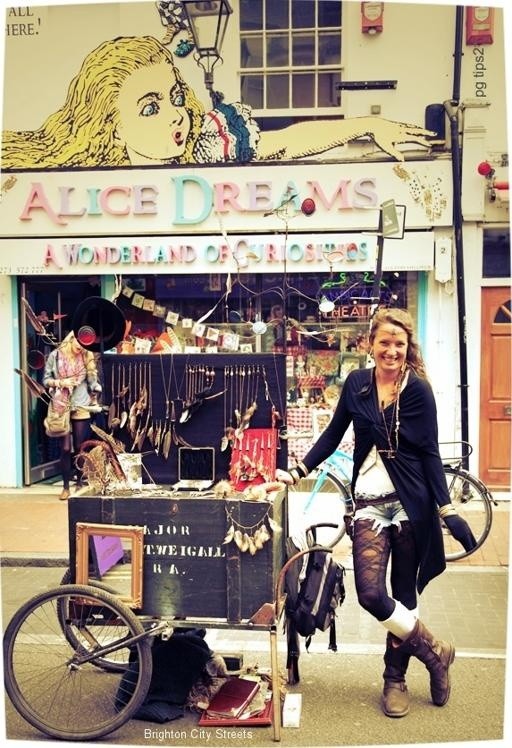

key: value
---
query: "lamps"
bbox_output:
[177,0,233,108]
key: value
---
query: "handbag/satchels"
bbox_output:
[43,401,71,438]
[285,525,344,635]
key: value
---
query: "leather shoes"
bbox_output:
[60,488,70,500]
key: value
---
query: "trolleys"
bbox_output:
[3,482,338,741]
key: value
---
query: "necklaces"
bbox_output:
[375,363,406,448]
[107,361,284,557]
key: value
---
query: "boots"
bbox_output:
[383,630,410,717]
[391,619,455,706]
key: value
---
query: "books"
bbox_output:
[206,678,259,719]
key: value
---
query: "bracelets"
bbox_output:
[296,461,309,478]
[438,502,460,520]
[59,378,64,389]
[289,468,300,483]
[52,380,56,387]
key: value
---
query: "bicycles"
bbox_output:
[287,410,498,562]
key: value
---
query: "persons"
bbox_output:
[42,329,102,500]
[275,306,477,718]
[2,35,438,169]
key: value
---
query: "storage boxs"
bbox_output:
[65,353,290,626]
